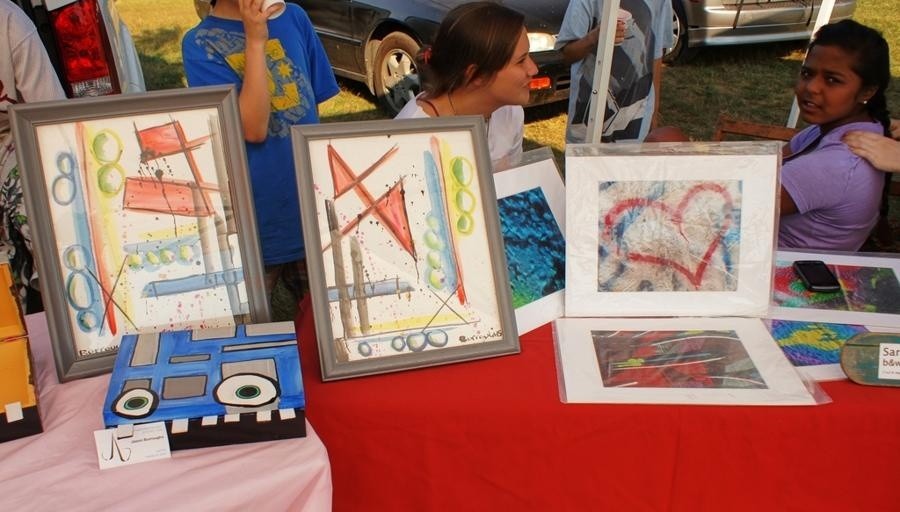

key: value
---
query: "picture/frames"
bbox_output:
[7,84,273,383]
[289,114,521,385]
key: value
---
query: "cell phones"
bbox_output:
[794,259,843,295]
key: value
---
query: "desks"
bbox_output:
[0,309,335,512]
[290,280,900,512]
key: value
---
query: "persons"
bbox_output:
[388,1,539,168]
[649,19,888,249]
[182,1,340,305]
[842,117,900,174]
[553,1,675,145]
[1,0,71,312]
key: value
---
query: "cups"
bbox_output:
[615,8,632,46]
[261,1,286,21]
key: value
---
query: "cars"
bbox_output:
[284,0,574,125]
[662,0,864,68]
